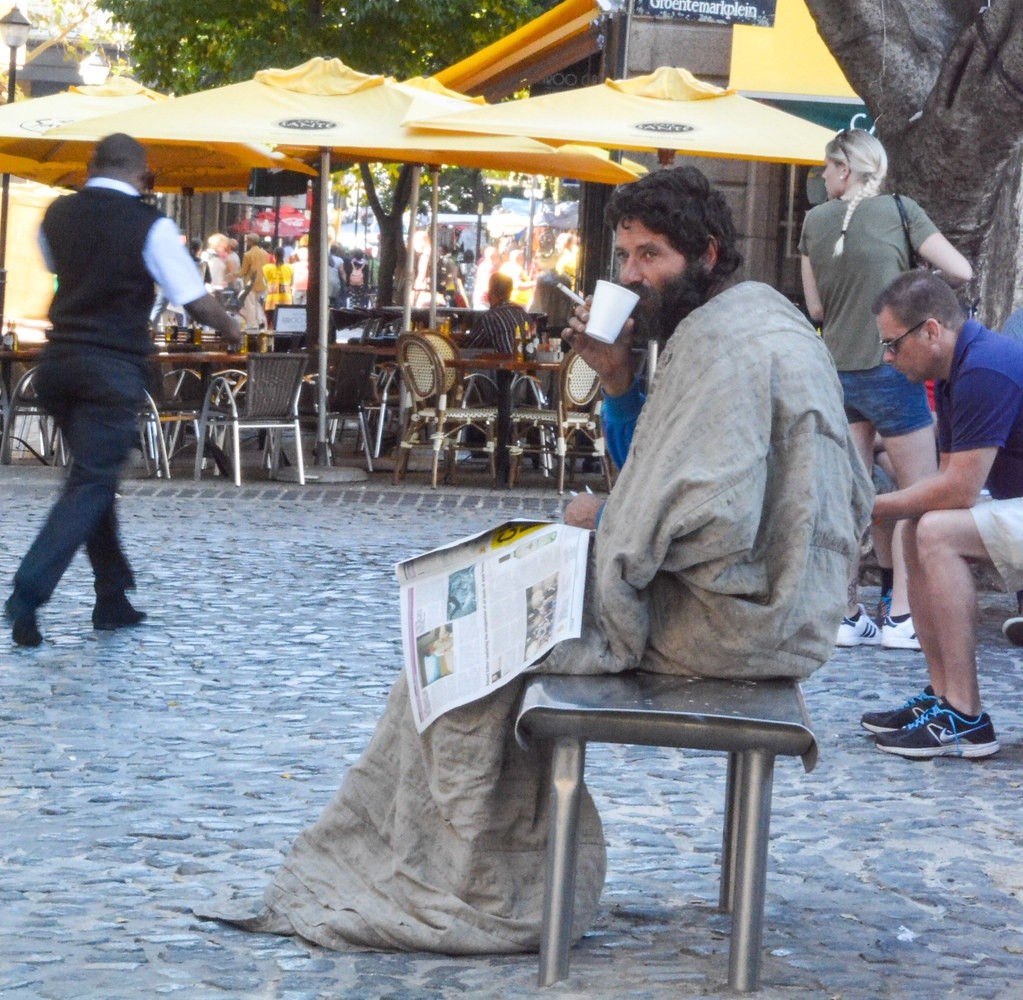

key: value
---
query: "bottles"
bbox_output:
[511,321,540,365]
[444,272,457,308]
[164,314,275,357]
[2,322,20,352]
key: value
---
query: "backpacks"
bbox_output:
[349,262,365,286]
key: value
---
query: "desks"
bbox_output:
[329,339,402,459]
[156,331,219,338]
[149,348,255,483]
[0,345,46,363]
[154,340,226,349]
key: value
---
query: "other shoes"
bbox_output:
[92,593,148,630]
[4,589,43,647]
[1002,617,1023,647]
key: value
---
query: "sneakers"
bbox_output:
[875,696,1001,757]
[861,685,941,733]
[835,603,881,646]
[877,589,921,649]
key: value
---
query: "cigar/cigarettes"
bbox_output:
[557,282,591,315]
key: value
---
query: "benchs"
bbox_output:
[513,671,820,992]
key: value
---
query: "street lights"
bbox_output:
[0,6,35,331]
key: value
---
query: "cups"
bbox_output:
[548,338,562,353]
[585,281,641,346]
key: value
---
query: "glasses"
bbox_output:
[879,318,940,354]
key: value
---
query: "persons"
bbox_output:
[7,134,243,645]
[281,167,873,953]
[861,266,1023,758]
[795,129,975,648]
[149,227,581,450]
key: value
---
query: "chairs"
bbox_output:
[0,329,616,496]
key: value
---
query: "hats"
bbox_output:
[246,233,261,242]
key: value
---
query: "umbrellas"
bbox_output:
[42,56,647,462]
[0,74,319,335]
[403,65,840,383]
